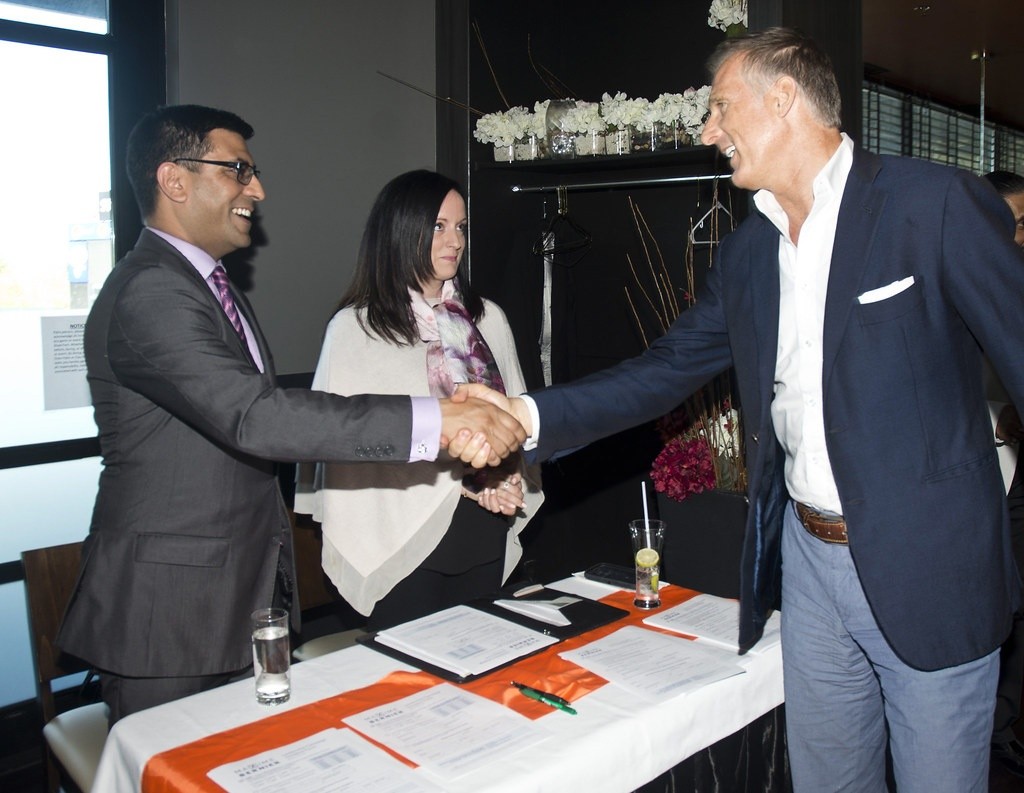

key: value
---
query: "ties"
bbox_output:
[213,266,247,347]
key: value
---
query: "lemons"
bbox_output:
[651,574,659,594]
[636,547,659,567]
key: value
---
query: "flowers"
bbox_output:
[470,99,602,138]
[603,87,720,124]
[650,431,762,501]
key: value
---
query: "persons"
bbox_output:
[981,171,1024,781]
[295,168,546,633]
[51,103,527,733]
[440,25,1024,793]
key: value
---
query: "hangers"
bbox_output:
[531,186,596,262]
[689,175,741,245]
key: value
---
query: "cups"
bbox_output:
[630,518,666,610]
[251,608,292,706]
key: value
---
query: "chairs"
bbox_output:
[289,511,382,659]
[22,538,111,792]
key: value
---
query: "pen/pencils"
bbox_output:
[510,681,578,714]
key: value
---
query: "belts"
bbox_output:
[796,503,847,543]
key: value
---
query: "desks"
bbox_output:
[93,566,787,793]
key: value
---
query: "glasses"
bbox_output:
[173,158,260,185]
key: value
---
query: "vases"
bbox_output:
[491,140,604,161]
[657,489,750,600]
[607,126,716,155]
